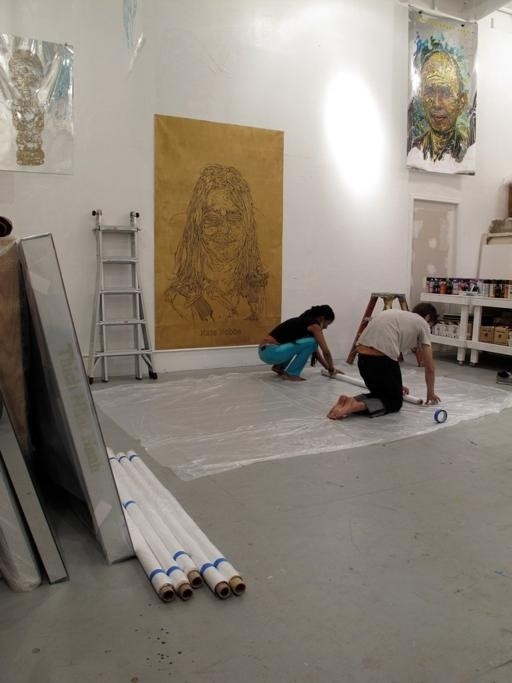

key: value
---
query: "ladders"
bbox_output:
[86,208,158,384]
[347,292,426,367]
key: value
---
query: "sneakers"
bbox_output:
[497,369,512,384]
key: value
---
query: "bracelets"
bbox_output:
[329,369,336,375]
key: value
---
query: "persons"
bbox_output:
[327,301,441,420]
[163,162,270,324]
[259,303,346,382]
[410,47,470,165]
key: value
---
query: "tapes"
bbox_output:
[435,409,447,423]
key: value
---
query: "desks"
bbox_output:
[418,292,512,367]
